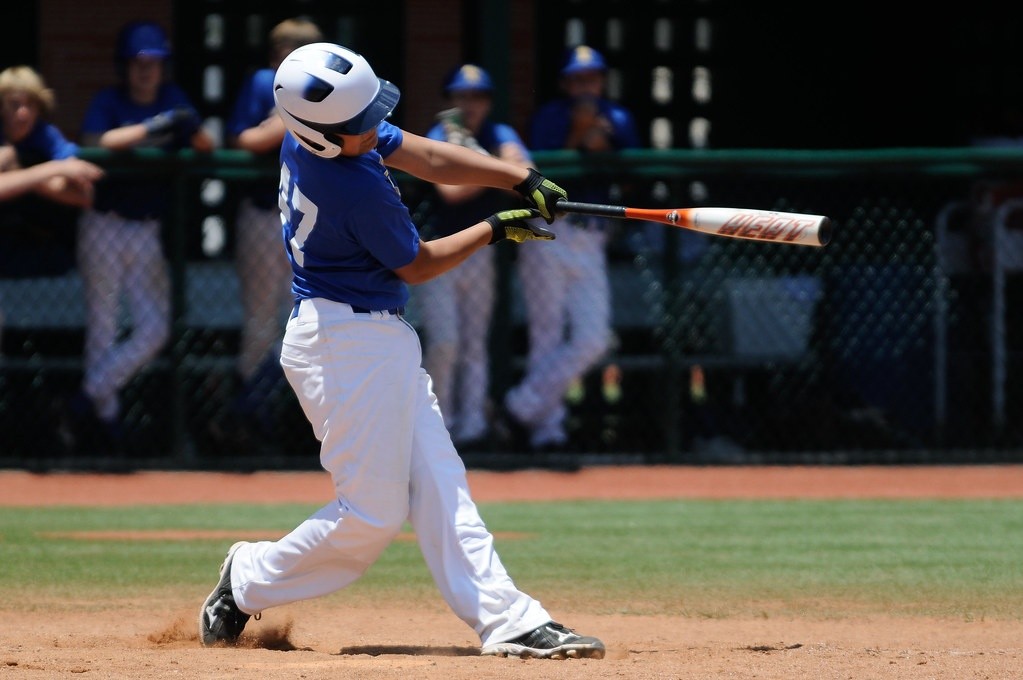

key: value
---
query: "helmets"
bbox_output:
[272,43,401,160]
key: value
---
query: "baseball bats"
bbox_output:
[555,200,834,247]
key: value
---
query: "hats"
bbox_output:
[115,20,173,56]
[444,64,497,90]
[562,46,605,74]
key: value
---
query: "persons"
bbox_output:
[69,23,202,452]
[500,46,633,477]
[238,20,325,384]
[416,64,534,447]
[0,66,103,203]
[198,43,608,659]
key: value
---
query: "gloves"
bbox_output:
[513,168,569,225]
[485,208,556,245]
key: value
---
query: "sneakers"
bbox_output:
[481,621,607,660]
[199,540,252,647]
